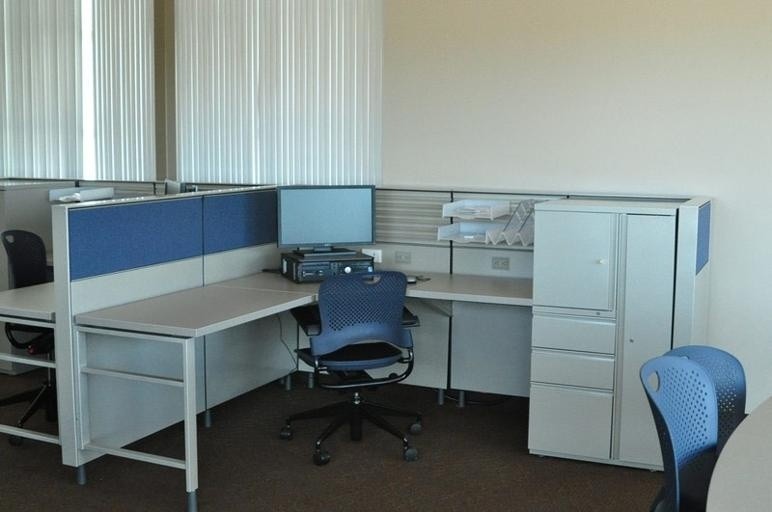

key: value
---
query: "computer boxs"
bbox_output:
[280,252,375,283]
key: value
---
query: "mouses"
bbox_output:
[407,274,417,283]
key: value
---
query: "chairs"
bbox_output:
[638,345,748,511]
[0,230,54,446]
[280,270,423,465]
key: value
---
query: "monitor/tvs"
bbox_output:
[276,184,376,257]
[164,178,185,195]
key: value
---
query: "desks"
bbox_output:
[704,398,772,512]
[1,280,60,448]
[211,268,531,317]
[76,285,316,492]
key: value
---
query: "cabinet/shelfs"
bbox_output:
[528,198,675,472]
